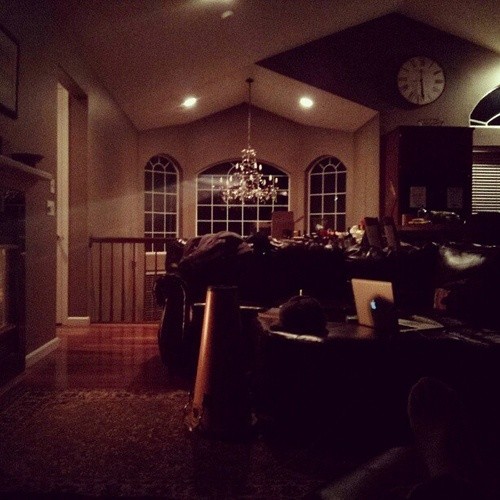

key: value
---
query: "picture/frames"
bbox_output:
[0,24,20,119]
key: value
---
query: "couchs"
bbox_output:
[152,232,500,364]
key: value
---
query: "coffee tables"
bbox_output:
[242,312,444,430]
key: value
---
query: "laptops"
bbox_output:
[351,279,444,329]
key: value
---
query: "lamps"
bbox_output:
[218,78,279,203]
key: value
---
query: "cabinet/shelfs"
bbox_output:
[379,125,476,235]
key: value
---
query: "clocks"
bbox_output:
[398,54,446,106]
